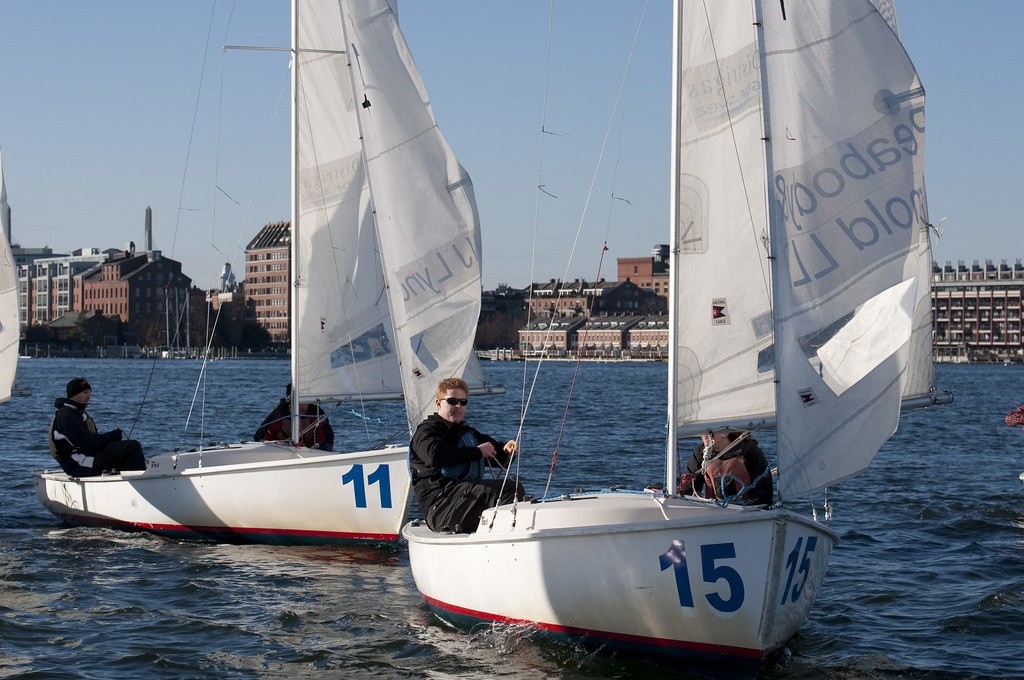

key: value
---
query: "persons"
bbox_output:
[252,383,333,452]
[648,428,773,511]
[51,378,145,477]
[406,376,524,534]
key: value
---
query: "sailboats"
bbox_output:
[402,0,935,668]
[31,0,506,549]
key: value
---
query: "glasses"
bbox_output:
[441,398,468,406]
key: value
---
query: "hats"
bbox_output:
[66,378,91,398]
